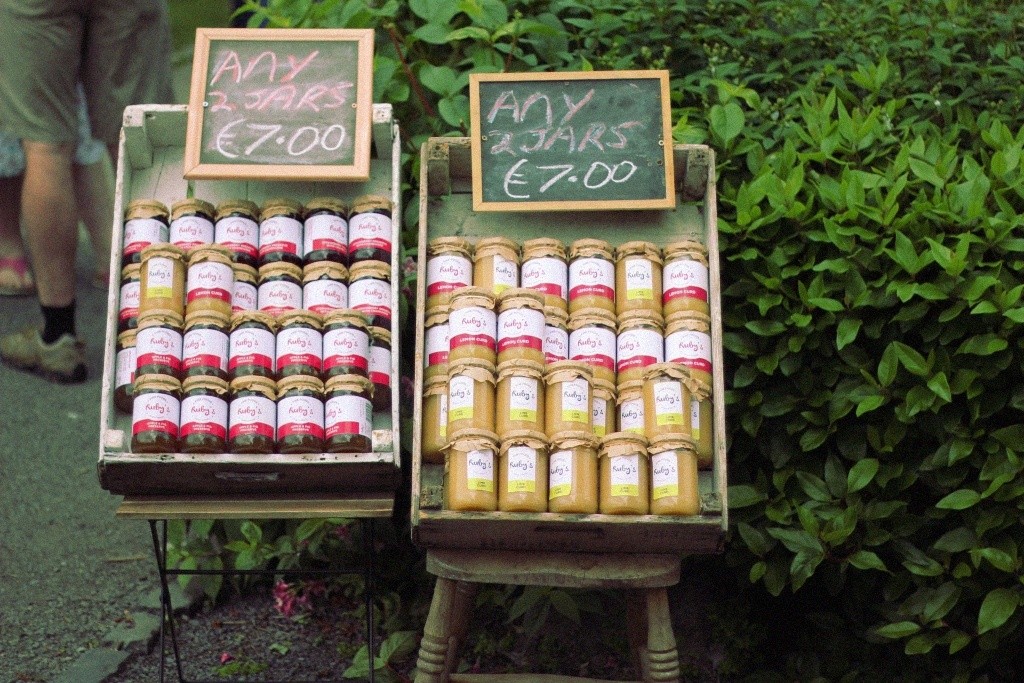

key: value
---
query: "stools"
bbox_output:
[414,547,682,683]
[117,492,394,683]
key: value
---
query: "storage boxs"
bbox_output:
[96,103,399,497]
[409,137,727,556]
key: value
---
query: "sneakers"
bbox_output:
[0,329,86,386]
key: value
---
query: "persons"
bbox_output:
[0,0,174,385]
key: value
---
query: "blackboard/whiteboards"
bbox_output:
[469,69,676,210]
[183,28,375,180]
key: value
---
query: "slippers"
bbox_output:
[0,245,35,296]
[93,270,111,291]
